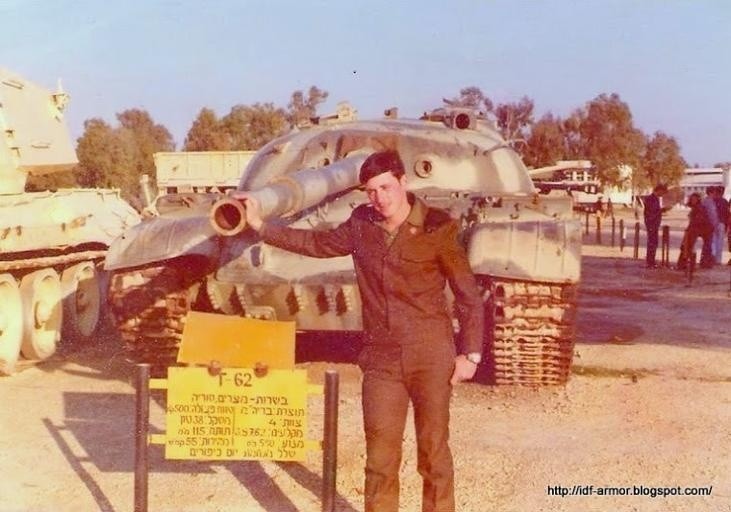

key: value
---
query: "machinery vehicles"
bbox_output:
[0,64,144,376]
[103,107,593,388]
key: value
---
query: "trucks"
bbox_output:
[142,150,258,219]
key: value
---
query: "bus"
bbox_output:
[532,180,602,211]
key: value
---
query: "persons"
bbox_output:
[229,146,492,511]
[643,182,731,274]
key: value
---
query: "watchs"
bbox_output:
[466,351,483,364]
